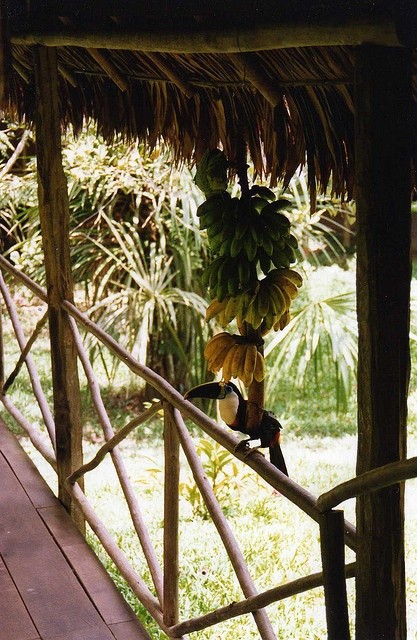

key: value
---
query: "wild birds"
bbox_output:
[184,381,289,478]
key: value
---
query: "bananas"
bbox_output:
[196,185,303,388]
